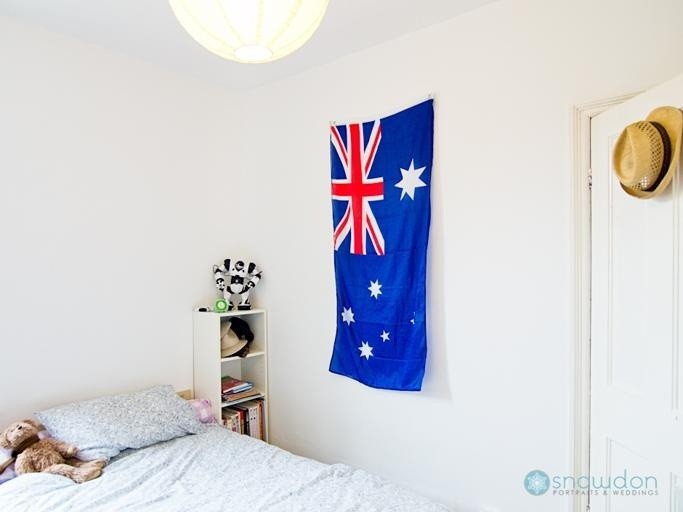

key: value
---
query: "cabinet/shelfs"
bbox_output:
[193,308,268,443]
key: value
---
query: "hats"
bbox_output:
[221,321,247,358]
[612,106,682,200]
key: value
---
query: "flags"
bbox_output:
[326,98,435,393]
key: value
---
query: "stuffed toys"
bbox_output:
[0,415,107,485]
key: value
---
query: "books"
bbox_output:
[220,376,267,442]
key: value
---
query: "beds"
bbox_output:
[0,389,458,512]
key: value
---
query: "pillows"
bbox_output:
[33,386,205,460]
